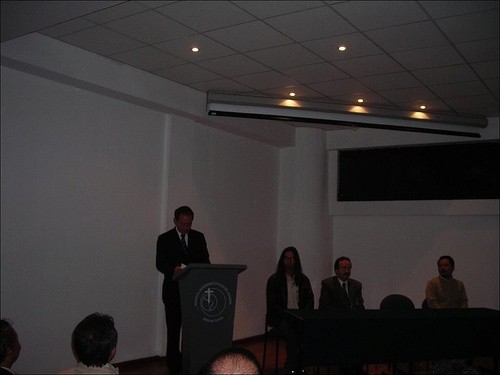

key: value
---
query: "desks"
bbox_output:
[285,306,500,375]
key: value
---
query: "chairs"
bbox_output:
[379,292,416,375]
[262,316,288,375]
[422,297,430,372]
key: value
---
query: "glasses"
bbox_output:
[284,256,296,260]
[338,266,352,270]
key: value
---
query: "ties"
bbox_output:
[342,283,350,311]
[180,233,187,255]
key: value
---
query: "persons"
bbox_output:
[319,257,368,375]
[55,313,120,375]
[426,256,469,309]
[0,319,22,375]
[155,206,212,371]
[203,347,262,375]
[266,246,314,375]
